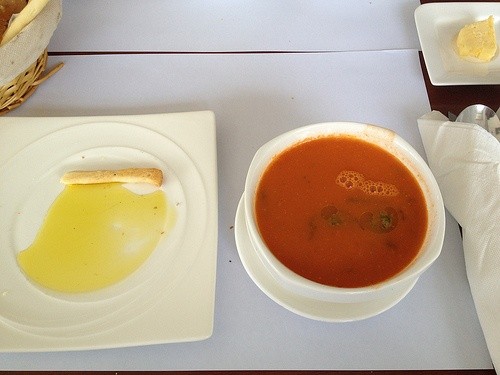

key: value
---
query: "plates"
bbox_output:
[414,1,500,89]
[233,192,420,322]
[0,109,220,351]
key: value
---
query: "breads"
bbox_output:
[60,168,164,186]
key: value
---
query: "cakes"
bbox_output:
[1,0,62,85]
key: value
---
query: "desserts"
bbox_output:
[457,15,496,63]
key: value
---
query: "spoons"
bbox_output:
[452,103,499,145]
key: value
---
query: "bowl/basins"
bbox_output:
[243,120,446,294]
